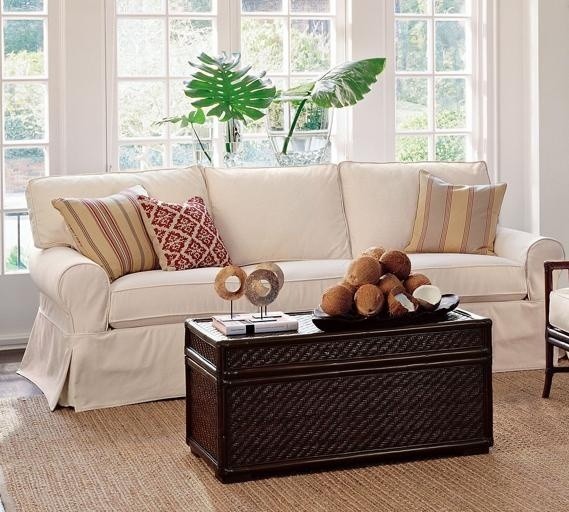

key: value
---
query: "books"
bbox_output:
[212,310,299,336]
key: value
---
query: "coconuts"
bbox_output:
[321,246,441,316]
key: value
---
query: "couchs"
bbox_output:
[16,160,568,414]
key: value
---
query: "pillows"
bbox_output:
[404,169,508,257]
[50,181,233,283]
